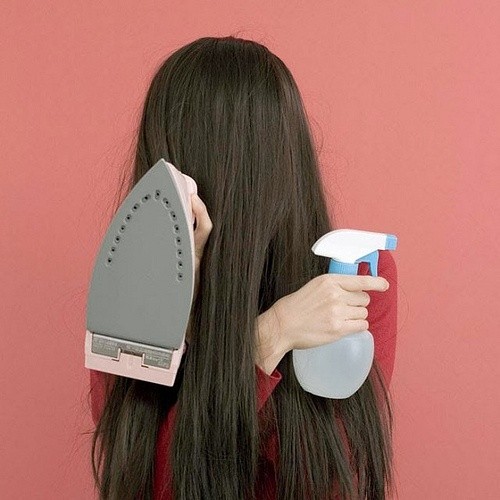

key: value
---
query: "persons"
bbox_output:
[88,41,400,500]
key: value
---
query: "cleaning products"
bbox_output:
[291,229,399,399]
